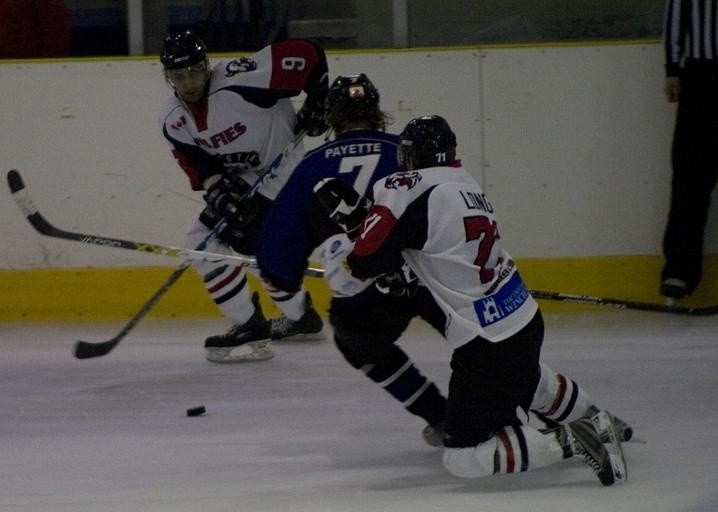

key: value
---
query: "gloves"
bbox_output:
[292,95,329,138]
[202,177,251,240]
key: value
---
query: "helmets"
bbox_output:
[325,72,386,133]
[396,114,458,171]
[160,30,212,91]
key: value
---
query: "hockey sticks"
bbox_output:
[74,133,314,359]
[6,168,325,279]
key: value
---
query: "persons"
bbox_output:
[659,0,718,298]
[255,76,450,448]
[323,114,633,489]
[156,31,330,350]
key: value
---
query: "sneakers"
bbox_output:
[660,262,691,297]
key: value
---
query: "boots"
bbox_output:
[204,291,272,349]
[267,291,324,342]
[533,403,633,488]
